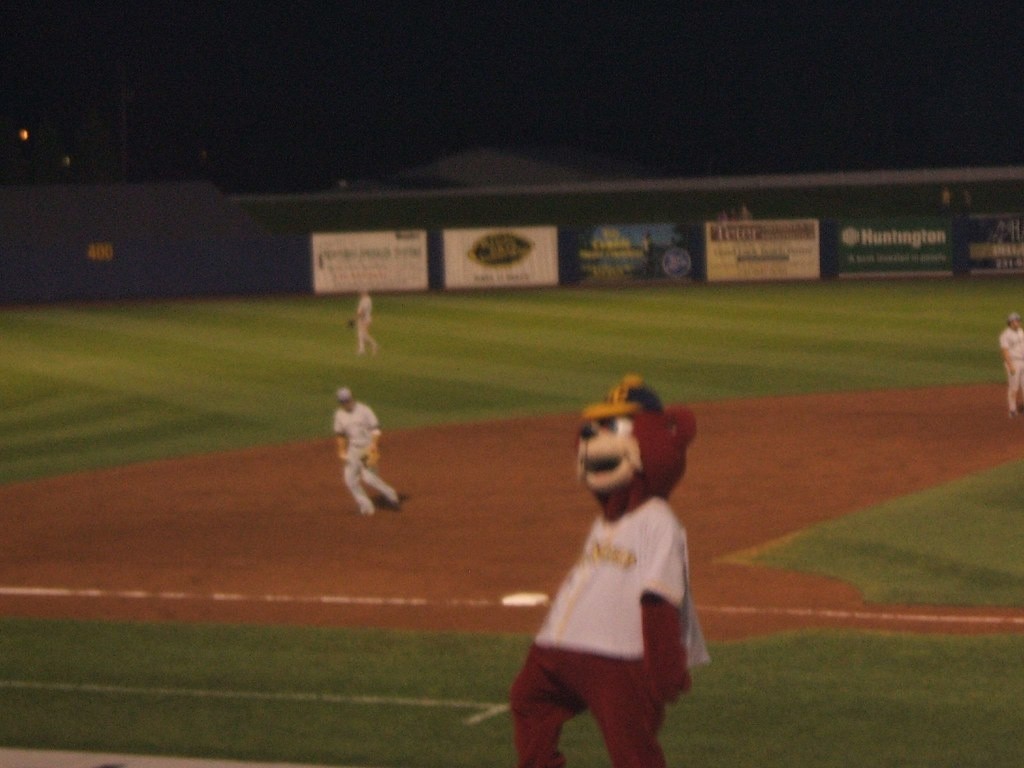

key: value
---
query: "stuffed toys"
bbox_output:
[509,373,714,768]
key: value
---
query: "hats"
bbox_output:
[1008,313,1020,321]
[336,387,352,400]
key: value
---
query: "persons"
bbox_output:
[355,287,377,357]
[333,386,399,517]
[1000,312,1024,419]
